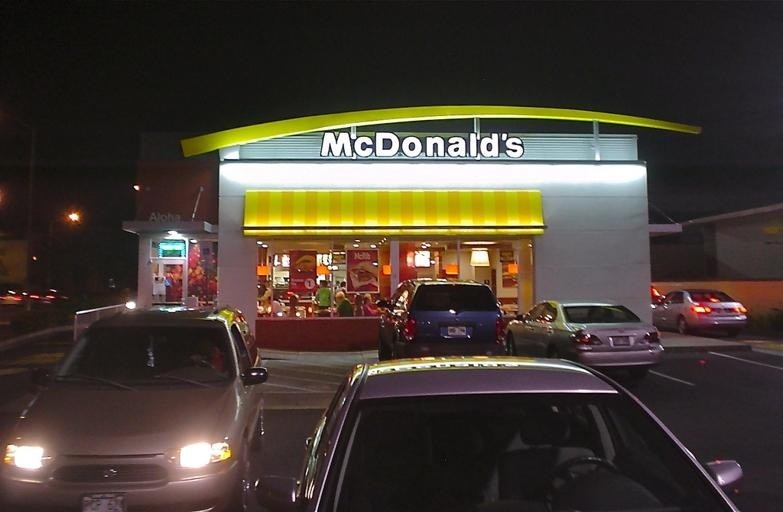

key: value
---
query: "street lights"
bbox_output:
[45,211,86,302]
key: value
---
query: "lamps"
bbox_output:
[470,248,490,266]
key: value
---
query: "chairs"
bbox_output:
[486,416,595,510]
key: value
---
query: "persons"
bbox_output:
[333,279,346,291]
[362,293,383,315]
[314,280,331,310]
[289,294,299,313]
[333,291,353,316]
[257,285,270,308]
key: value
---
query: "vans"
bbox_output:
[0,302,269,512]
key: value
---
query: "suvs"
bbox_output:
[375,278,508,361]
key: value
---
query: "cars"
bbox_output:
[0,288,63,307]
[651,287,747,337]
[255,358,746,512]
[651,285,665,310]
[502,298,664,380]
